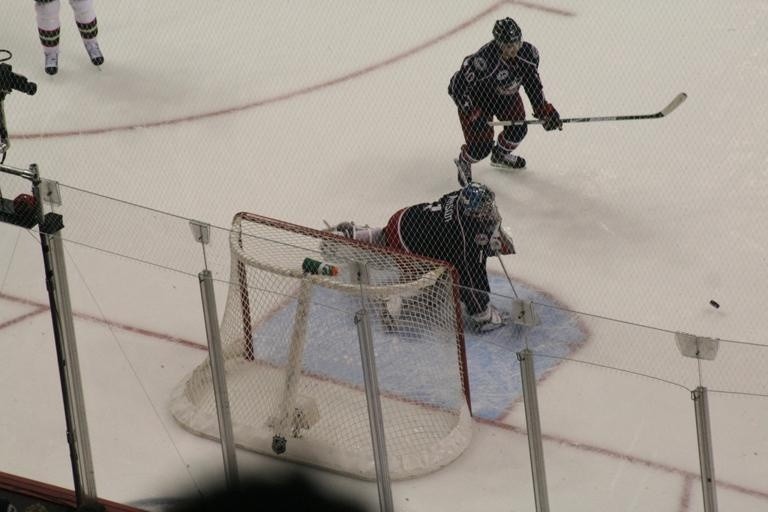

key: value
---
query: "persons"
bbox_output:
[36,0,104,75]
[301,180,512,336]
[447,15,565,188]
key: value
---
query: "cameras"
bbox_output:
[0,63,37,99]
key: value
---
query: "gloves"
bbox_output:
[534,104,563,130]
[467,109,491,132]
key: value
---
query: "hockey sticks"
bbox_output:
[486,92,688,126]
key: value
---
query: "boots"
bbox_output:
[491,148,526,168]
[457,146,472,186]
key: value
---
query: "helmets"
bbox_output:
[491,17,522,48]
[460,181,497,222]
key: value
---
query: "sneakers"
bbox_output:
[42,45,58,73]
[84,37,103,63]
